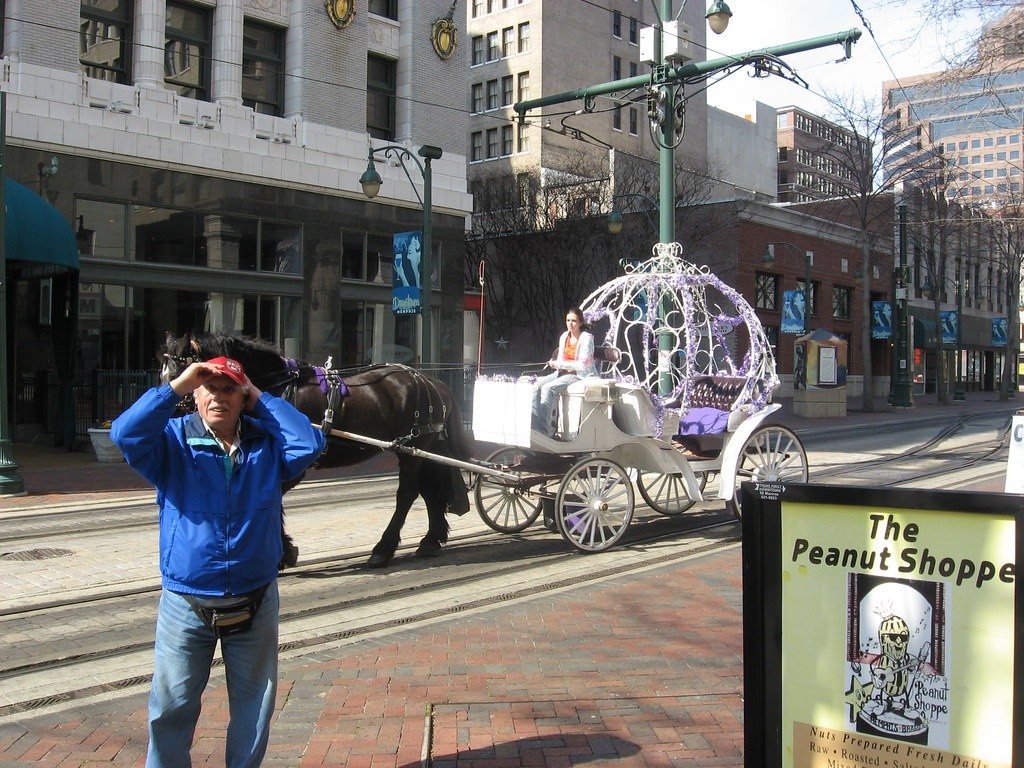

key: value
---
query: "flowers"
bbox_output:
[94,417,112,430]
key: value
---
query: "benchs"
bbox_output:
[615,381,679,445]
[522,346,623,437]
[662,376,770,455]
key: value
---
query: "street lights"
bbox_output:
[629,0,733,396]
[360,142,443,372]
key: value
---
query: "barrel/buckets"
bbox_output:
[872,375,891,412]
[845,374,864,411]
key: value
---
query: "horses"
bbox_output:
[158,330,473,570]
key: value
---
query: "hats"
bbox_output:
[207,356,246,385]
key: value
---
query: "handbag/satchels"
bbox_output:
[192,594,261,637]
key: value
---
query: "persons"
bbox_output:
[110,356,328,768]
[531,308,599,438]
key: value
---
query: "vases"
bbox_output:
[87,427,124,462]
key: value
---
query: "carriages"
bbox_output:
[157,240,809,569]
